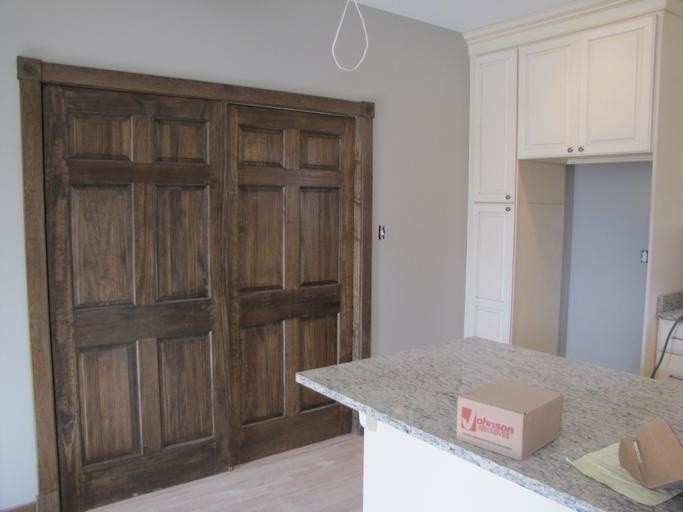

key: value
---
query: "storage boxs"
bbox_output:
[454,377,565,459]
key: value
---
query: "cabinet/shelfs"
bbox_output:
[515,11,683,164]
[463,53,565,357]
[654,319,682,387]
